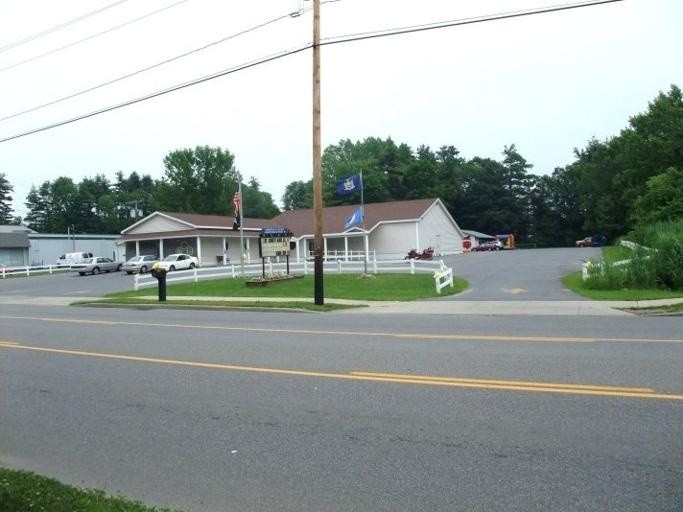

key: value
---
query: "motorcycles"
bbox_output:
[405,247,434,261]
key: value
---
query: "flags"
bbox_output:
[343,207,360,228]
[232,183,239,231]
[334,172,360,196]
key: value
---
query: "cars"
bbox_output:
[120,255,161,275]
[55,252,93,268]
[151,252,198,272]
[574,236,593,248]
[470,241,502,252]
[70,256,123,276]
